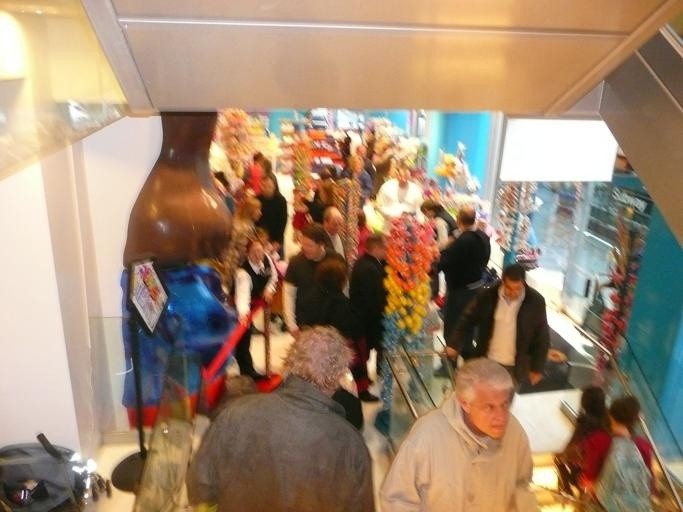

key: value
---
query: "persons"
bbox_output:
[183,127,655,511]
